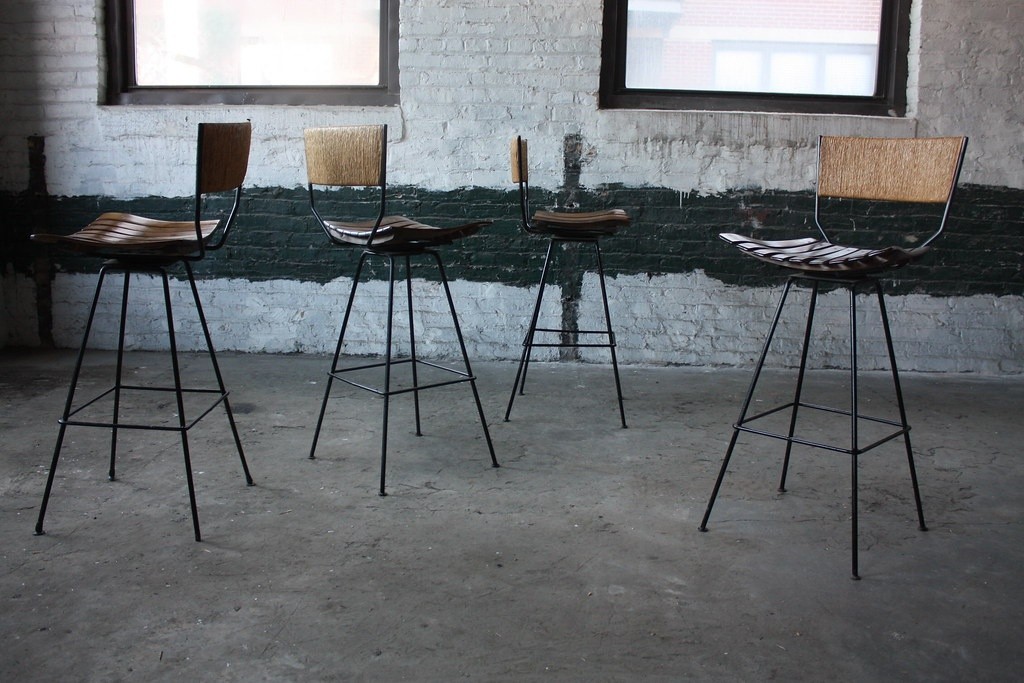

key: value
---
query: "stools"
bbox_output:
[295,124,504,496]
[698,135,969,580]
[504,136,631,428]
[25,122,258,541]
